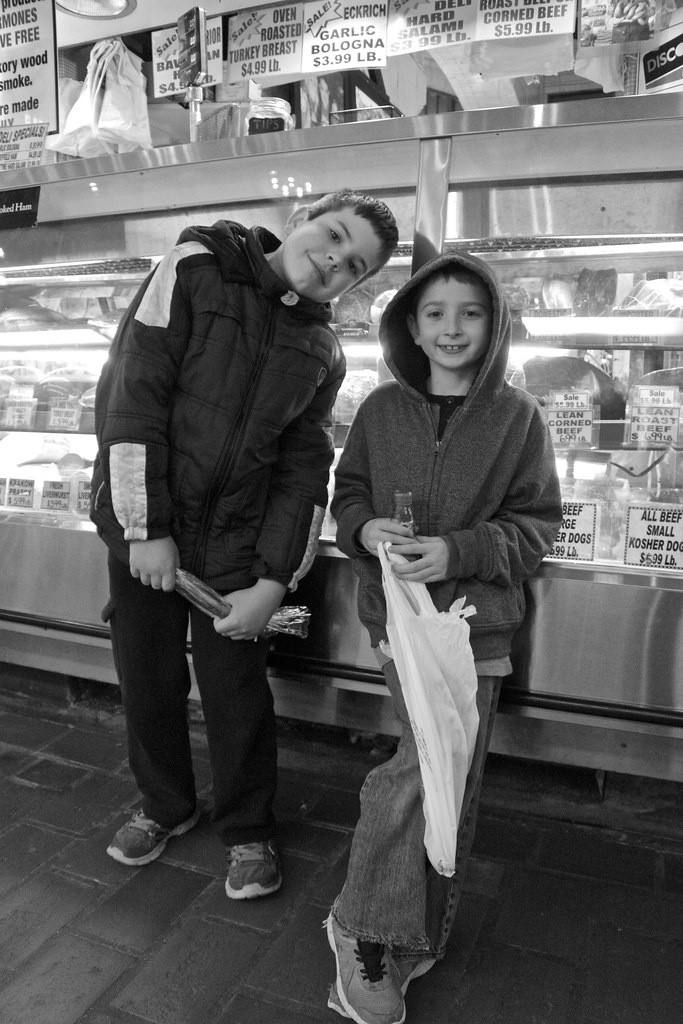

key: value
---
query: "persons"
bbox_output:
[327,246,564,1024]
[87,189,400,900]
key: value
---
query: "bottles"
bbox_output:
[244,97,295,135]
[389,489,422,562]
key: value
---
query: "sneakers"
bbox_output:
[225,840,283,900]
[328,937,436,1018]
[107,806,199,866]
[326,908,407,1024]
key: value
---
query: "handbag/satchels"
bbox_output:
[377,540,478,877]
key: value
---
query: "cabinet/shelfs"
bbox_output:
[0,92,683,785]
